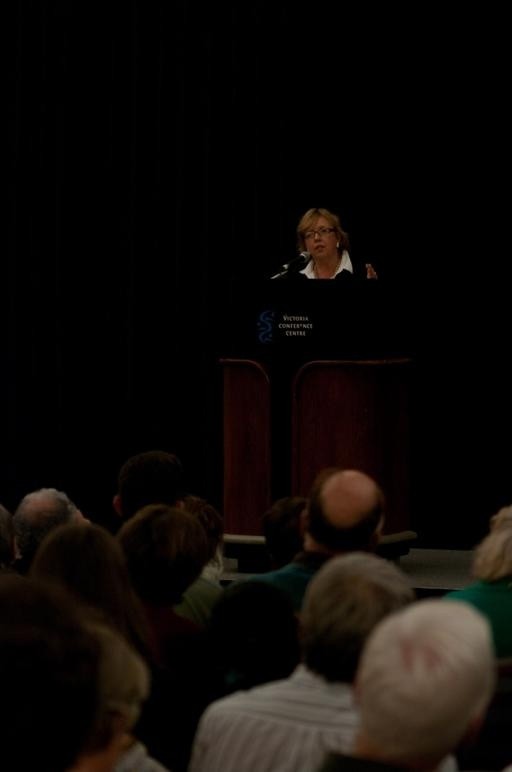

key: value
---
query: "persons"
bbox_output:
[0,450,512,770]
[285,206,380,279]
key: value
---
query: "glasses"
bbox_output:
[303,228,336,240]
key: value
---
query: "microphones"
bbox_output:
[282,251,310,270]
